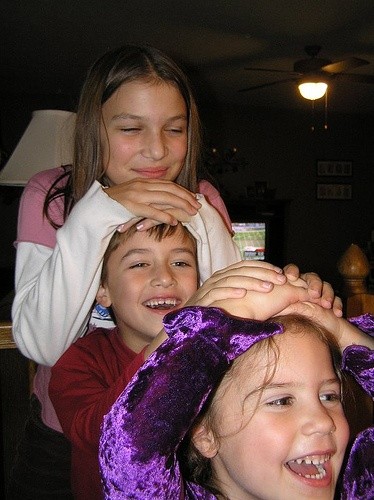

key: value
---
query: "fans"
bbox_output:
[238,45,374,92]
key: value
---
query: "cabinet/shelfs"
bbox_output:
[232,200,288,266]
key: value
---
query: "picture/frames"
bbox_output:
[315,159,354,201]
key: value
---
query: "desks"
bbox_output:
[0,287,39,408]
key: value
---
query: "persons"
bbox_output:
[12,43,243,499]
[99,280,374,500]
[48,217,342,500]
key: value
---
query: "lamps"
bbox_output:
[0,109,79,249]
